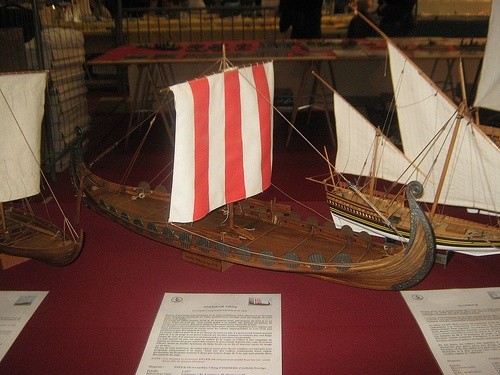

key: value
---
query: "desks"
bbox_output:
[82,35,488,147]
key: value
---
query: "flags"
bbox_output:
[169,62,274,224]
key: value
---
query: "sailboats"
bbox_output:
[68,44,436,290]
[305,16,500,260]
[2,69,84,269]
[457,1,499,151]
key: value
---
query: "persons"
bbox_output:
[378,0,418,37]
[81,0,112,20]
[278,0,323,39]
[158,0,180,19]
[347,0,374,37]
